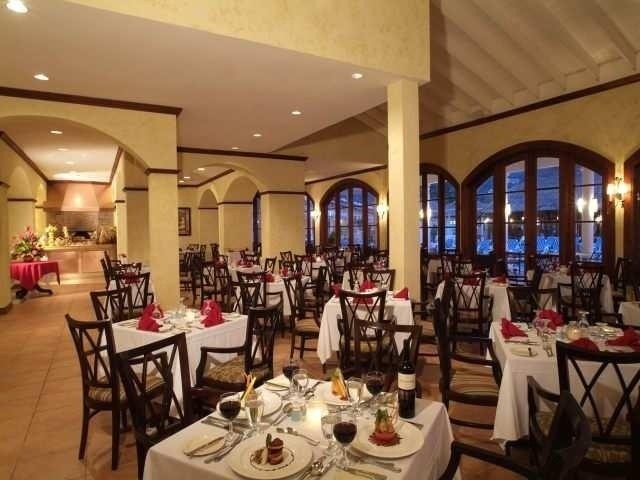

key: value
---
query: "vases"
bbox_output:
[23,255,32,261]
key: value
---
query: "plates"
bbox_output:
[228,432,313,480]
[158,324,172,332]
[349,420,425,459]
[216,389,282,420]
[511,347,537,356]
[315,380,373,406]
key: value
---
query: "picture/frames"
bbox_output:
[178,207,192,236]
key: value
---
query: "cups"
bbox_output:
[177,304,185,318]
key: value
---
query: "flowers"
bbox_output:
[8,225,42,258]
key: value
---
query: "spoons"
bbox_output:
[276,426,320,445]
[270,403,293,426]
[308,382,320,392]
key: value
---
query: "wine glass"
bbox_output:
[280,356,308,411]
[533,307,616,358]
[320,370,386,470]
[244,394,264,437]
[151,302,161,320]
[220,391,242,442]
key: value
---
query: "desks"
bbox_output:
[7,261,60,298]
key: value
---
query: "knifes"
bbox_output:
[528,348,532,356]
[183,416,251,462]
[265,382,289,388]
[299,451,402,480]
[504,339,536,344]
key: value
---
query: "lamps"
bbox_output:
[606,178,630,208]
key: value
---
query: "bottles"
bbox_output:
[398,339,417,418]
